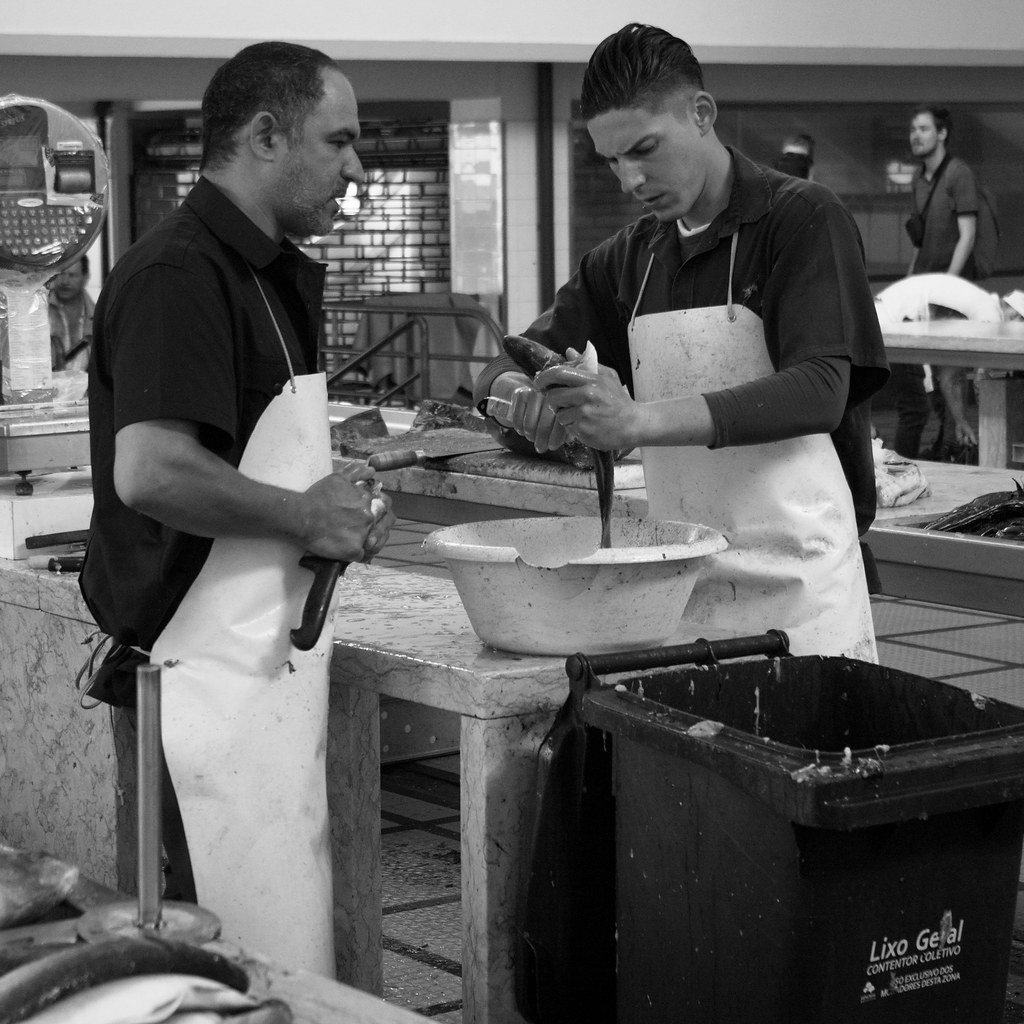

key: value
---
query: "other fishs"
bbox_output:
[503,334,614,549]
[0,843,78,927]
[2,938,249,1021]
[924,478,1024,531]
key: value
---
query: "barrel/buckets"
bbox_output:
[565,628,1024,1022]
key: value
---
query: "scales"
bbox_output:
[1,92,110,496]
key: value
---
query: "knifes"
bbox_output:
[367,444,507,472]
[477,396,513,429]
[289,550,350,651]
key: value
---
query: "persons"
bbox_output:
[473,22,890,662]
[778,133,814,178]
[904,104,981,278]
[49,255,94,370]
[874,272,1023,458]
[79,41,395,981]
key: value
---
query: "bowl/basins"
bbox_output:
[423,517,731,657]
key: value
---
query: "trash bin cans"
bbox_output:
[513,628,1024,1023]
